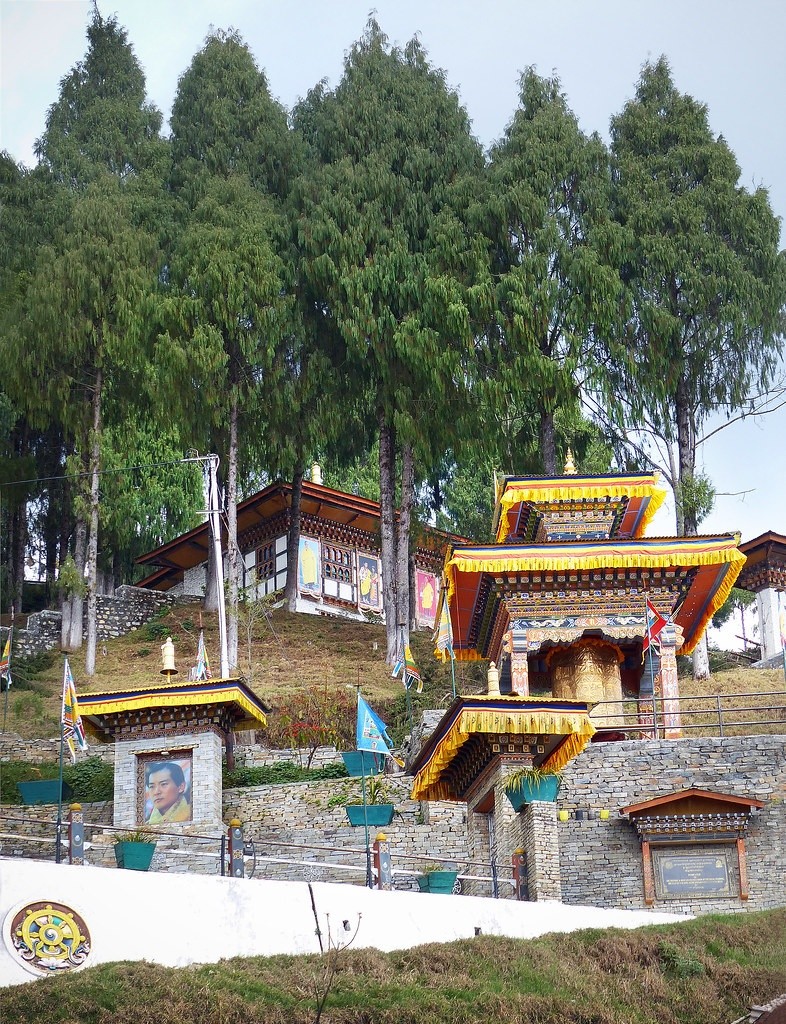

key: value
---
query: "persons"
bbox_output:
[145,763,191,824]
[421,574,434,620]
[302,540,316,589]
[360,562,378,607]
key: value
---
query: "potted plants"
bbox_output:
[414,863,458,894]
[326,774,405,826]
[109,825,157,872]
[495,762,573,814]
[16,767,75,805]
[337,728,386,777]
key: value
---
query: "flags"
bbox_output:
[357,696,405,767]
[392,634,423,693]
[195,633,213,681]
[0,624,12,684]
[63,660,88,763]
[642,597,669,662]
[430,598,456,658]
